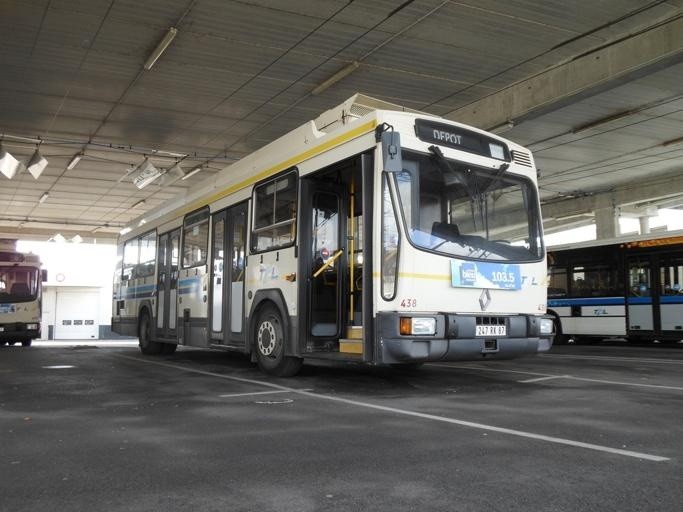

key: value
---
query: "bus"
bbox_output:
[111,93,557,377]
[0,250,47,347]
[538,229,683,344]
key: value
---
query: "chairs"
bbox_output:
[430,221,459,246]
[10,282,29,296]
